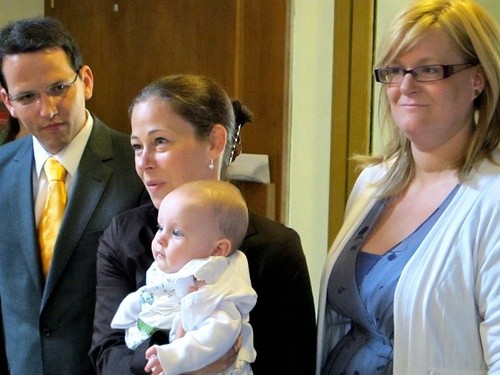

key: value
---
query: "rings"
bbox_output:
[232,346,237,353]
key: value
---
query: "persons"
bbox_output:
[0,16,153,375]
[111,180,258,375]
[315,1,500,375]
[88,73,317,375]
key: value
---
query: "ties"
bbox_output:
[36,157,67,282]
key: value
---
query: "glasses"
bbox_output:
[374,60,480,84]
[7,73,78,108]
